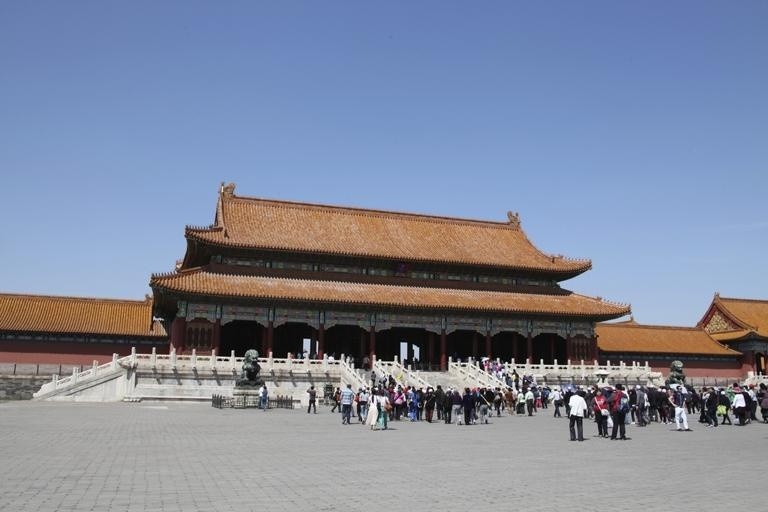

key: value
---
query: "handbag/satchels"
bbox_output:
[333,397,336,401]
[602,409,610,417]
[715,405,727,416]
[376,399,381,411]
[559,400,564,407]
[384,404,394,411]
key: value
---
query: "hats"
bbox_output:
[358,383,548,393]
[702,383,756,395]
[554,384,668,393]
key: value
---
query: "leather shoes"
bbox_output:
[677,428,692,431]
[497,410,537,417]
[610,436,631,440]
[570,438,584,441]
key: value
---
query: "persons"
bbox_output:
[483,360,534,390]
[363,356,369,371]
[256,384,265,409]
[560,384,699,441]
[331,375,425,430]
[698,383,768,427]
[307,386,317,413]
[425,385,561,425]
[262,386,268,412]
[293,352,354,364]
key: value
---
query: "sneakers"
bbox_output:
[630,419,676,427]
[342,419,350,425]
[407,418,489,425]
[359,418,365,424]
[599,435,610,438]
[699,418,768,427]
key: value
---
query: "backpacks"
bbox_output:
[617,397,629,415]
